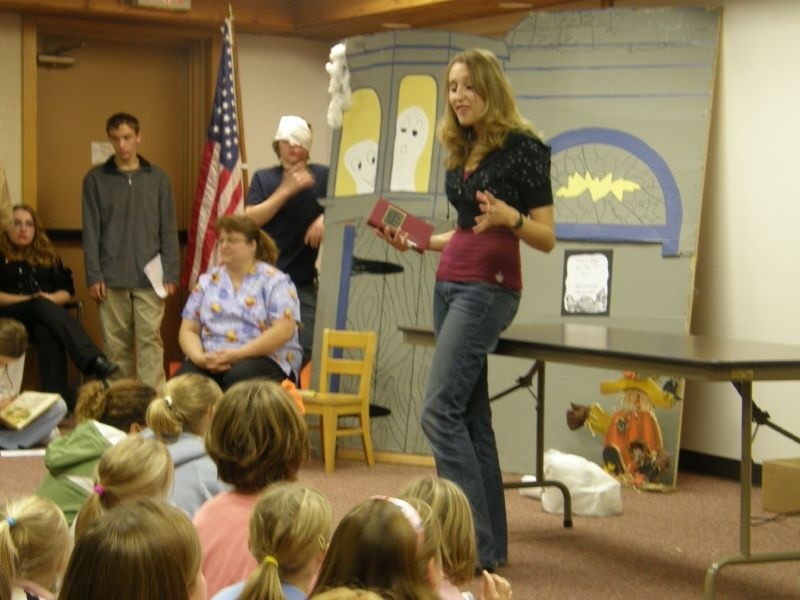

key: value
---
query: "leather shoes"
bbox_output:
[91,356,120,380]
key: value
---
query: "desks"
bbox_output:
[395,325,800,600]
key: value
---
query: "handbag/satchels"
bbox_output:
[541,448,622,517]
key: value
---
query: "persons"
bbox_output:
[298,491,475,600]
[372,46,558,575]
[385,472,515,599]
[134,371,235,525]
[0,318,67,449]
[80,109,181,405]
[0,494,67,600]
[0,201,121,416]
[54,495,209,600]
[239,112,332,389]
[176,213,305,394]
[73,433,177,547]
[31,378,158,525]
[185,376,315,600]
[211,489,334,600]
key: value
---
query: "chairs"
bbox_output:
[299,329,377,472]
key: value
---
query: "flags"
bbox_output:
[183,17,249,311]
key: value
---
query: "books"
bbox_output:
[367,199,434,254]
[0,388,60,430]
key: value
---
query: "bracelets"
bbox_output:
[510,213,526,232]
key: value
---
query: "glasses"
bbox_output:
[219,234,247,245]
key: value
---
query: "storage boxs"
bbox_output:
[760,460,800,512]
